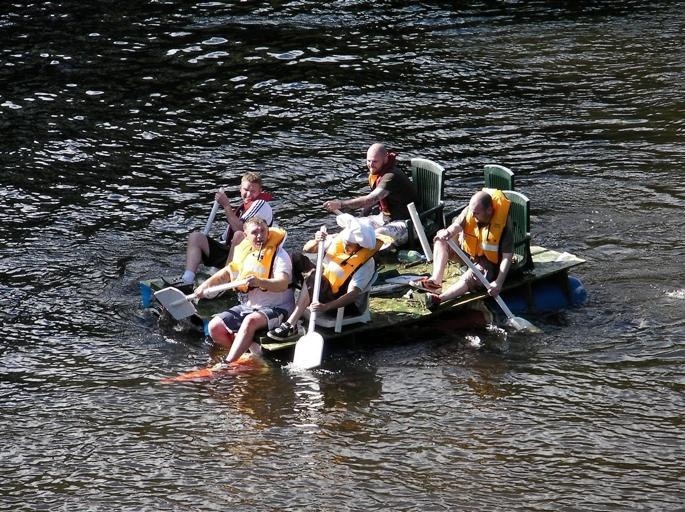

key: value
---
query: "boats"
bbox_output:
[134,240,595,372]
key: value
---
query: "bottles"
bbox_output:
[396,249,427,263]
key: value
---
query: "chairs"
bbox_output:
[503,190,535,277]
[484,164,515,190]
[406,158,446,250]
[304,268,379,332]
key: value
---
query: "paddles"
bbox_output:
[154,282,251,320]
[448,239,537,331]
[292,227,324,371]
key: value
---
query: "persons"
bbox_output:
[408,191,515,309]
[323,142,424,251]
[165,171,273,295]
[267,222,379,341]
[194,217,294,371]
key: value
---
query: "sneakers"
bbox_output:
[423,293,442,309]
[164,272,194,294]
[267,322,299,342]
[409,279,442,295]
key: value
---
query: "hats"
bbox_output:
[336,213,376,250]
